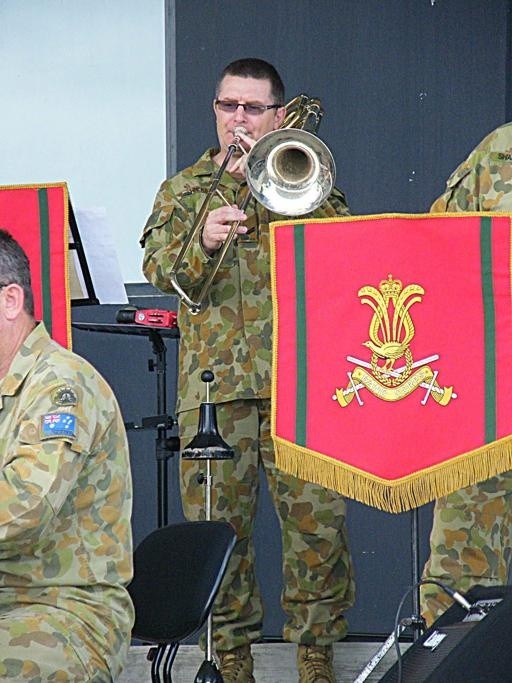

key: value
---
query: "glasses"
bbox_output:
[215,98,279,115]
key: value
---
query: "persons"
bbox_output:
[0,232,136,683]
[144,59,359,680]
[417,114,512,634]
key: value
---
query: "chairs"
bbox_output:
[119,521,239,682]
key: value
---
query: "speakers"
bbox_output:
[377,584,512,683]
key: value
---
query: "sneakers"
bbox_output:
[297,642,338,682]
[216,645,257,682]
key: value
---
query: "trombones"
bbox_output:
[167,94,337,316]
